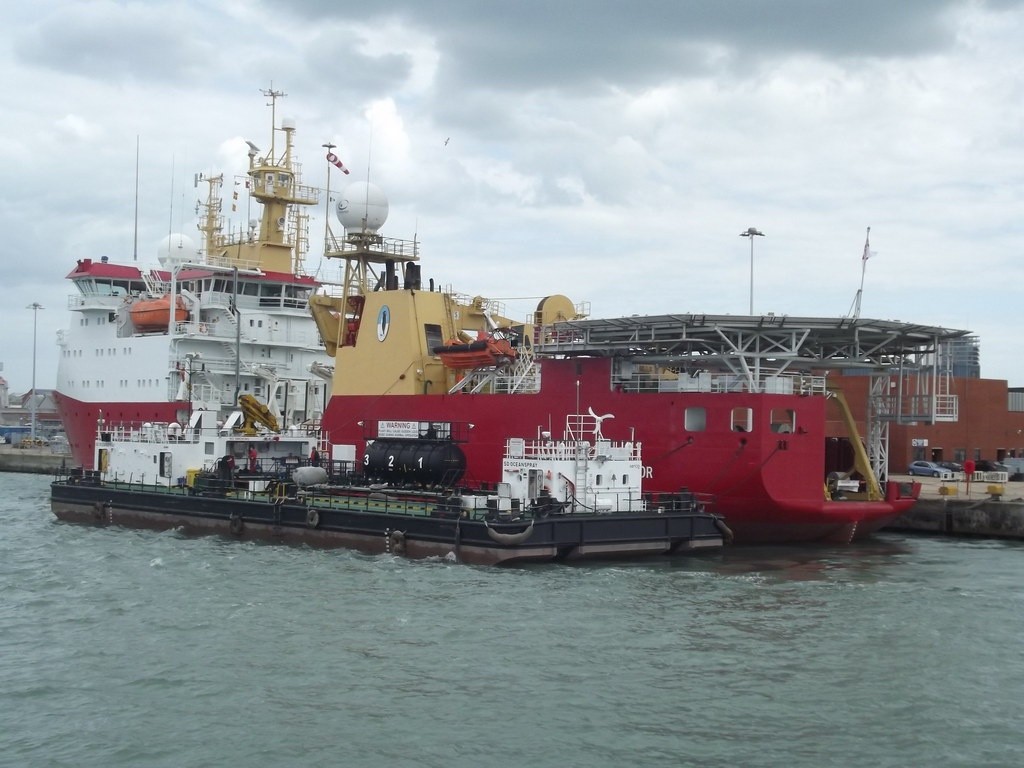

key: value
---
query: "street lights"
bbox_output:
[740,228,766,316]
[25,301,46,447]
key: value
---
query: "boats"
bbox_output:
[52,381,737,570]
[129,292,191,332]
[433,330,518,370]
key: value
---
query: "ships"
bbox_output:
[51,78,922,548]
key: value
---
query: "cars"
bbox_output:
[35,435,50,447]
[906,461,953,479]
[0,434,6,443]
[50,435,70,446]
[975,460,1010,473]
[936,461,964,472]
[3,432,13,444]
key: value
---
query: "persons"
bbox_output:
[248,446,257,472]
[310,446,320,467]
[226,455,235,487]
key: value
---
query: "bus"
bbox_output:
[0,422,45,445]
